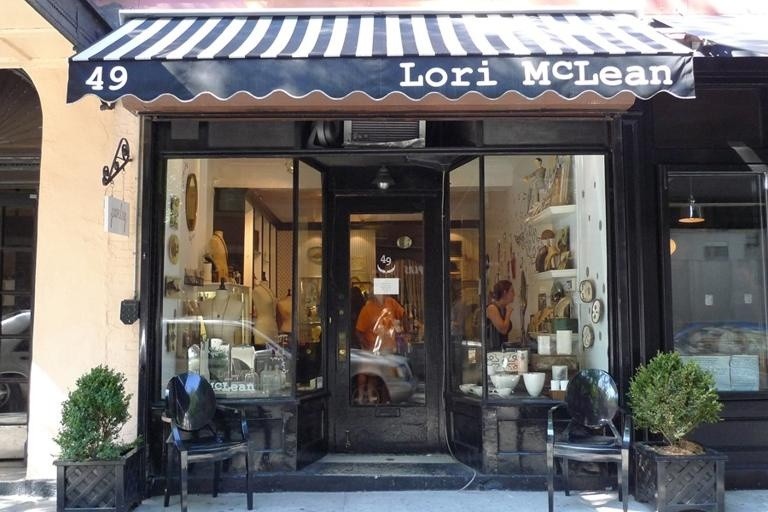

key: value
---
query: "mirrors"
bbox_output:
[183,172,198,232]
[394,233,413,251]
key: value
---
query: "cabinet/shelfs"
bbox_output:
[520,202,578,342]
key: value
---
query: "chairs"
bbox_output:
[547,367,634,511]
[163,369,254,512]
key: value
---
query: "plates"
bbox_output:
[169,236,179,263]
[581,325,594,348]
[579,279,593,304]
[590,298,602,324]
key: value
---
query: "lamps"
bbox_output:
[677,175,707,225]
[370,164,397,190]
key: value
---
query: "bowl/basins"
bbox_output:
[491,374,521,390]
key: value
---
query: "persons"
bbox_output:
[199,290,246,346]
[356,278,415,405]
[484,280,514,347]
[535,229,554,271]
[521,157,546,199]
[252,278,281,345]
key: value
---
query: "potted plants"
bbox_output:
[624,347,730,511]
[52,361,150,512]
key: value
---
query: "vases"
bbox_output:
[535,334,552,355]
[522,370,547,400]
[489,372,520,396]
[555,328,573,358]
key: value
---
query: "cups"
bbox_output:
[551,365,568,401]
[202,263,212,283]
[524,373,545,397]
[537,335,550,354]
[458,383,512,397]
[556,329,573,356]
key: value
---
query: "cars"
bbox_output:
[0,309,32,411]
[163,314,418,410]
[672,321,767,353]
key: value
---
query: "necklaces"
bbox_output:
[259,283,275,302]
[213,297,229,319]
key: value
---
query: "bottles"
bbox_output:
[527,315,536,336]
[234,271,239,284]
[260,365,285,390]
[223,368,259,392]
